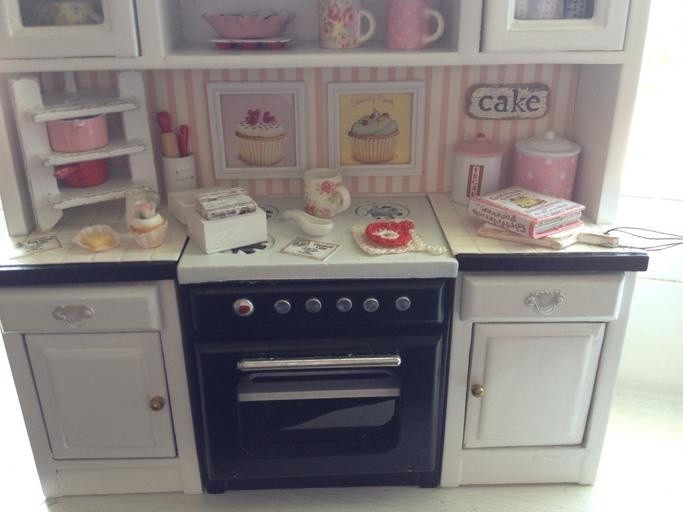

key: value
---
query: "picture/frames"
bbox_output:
[327,79,425,178]
[207,80,307,181]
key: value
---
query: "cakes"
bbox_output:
[81,232,112,249]
[127,199,169,248]
[348,106,399,164]
[235,109,286,167]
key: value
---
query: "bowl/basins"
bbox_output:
[202,11,297,38]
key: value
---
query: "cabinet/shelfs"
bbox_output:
[439,269,636,490]
[1,281,205,494]
[0,1,647,237]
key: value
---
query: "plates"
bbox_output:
[206,36,295,49]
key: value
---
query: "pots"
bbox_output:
[45,113,107,153]
[51,158,109,188]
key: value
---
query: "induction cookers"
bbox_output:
[180,195,460,341]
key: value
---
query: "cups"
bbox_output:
[48,0,101,27]
[318,1,375,51]
[383,1,445,51]
[516,0,585,20]
[161,152,197,195]
[130,219,168,249]
[303,168,350,218]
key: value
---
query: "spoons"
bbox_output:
[280,208,333,237]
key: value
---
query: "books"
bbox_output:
[468,185,586,240]
[478,223,593,248]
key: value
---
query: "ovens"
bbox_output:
[186,336,448,494]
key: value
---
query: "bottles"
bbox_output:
[452,134,506,207]
[513,131,583,200]
